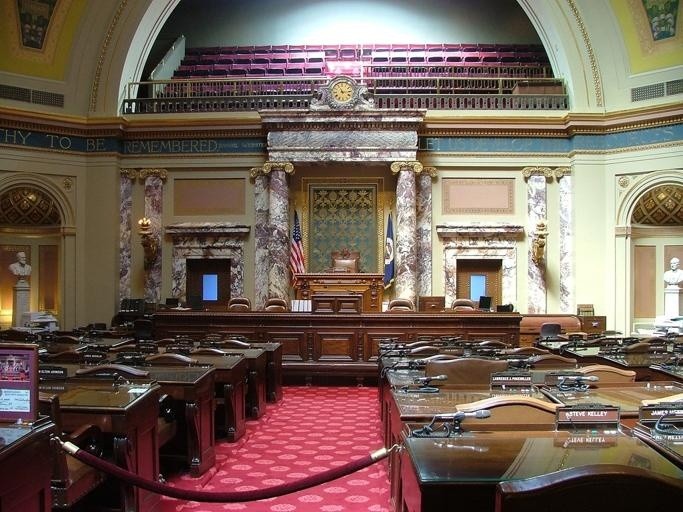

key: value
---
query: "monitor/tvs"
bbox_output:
[0,343,38,423]
[477,296,491,310]
[166,298,178,307]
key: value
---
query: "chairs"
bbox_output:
[264,297,287,312]
[388,298,413,310]
[228,297,251,311]
[163,43,553,108]
[0,324,683,512]
[451,299,475,309]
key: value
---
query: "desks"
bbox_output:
[511,81,564,104]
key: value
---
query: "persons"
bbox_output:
[663,257,683,285]
[8,251,32,280]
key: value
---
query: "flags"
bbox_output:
[289,210,305,274]
[381,214,395,291]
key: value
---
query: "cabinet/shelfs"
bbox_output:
[419,296,445,311]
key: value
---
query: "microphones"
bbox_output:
[666,410,683,416]
[391,343,407,346]
[434,442,489,452]
[558,376,599,382]
[397,359,432,364]
[669,356,683,360]
[415,375,449,381]
[507,357,536,362]
[390,349,411,353]
[435,410,492,418]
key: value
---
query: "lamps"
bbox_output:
[531,221,549,267]
[137,218,158,259]
